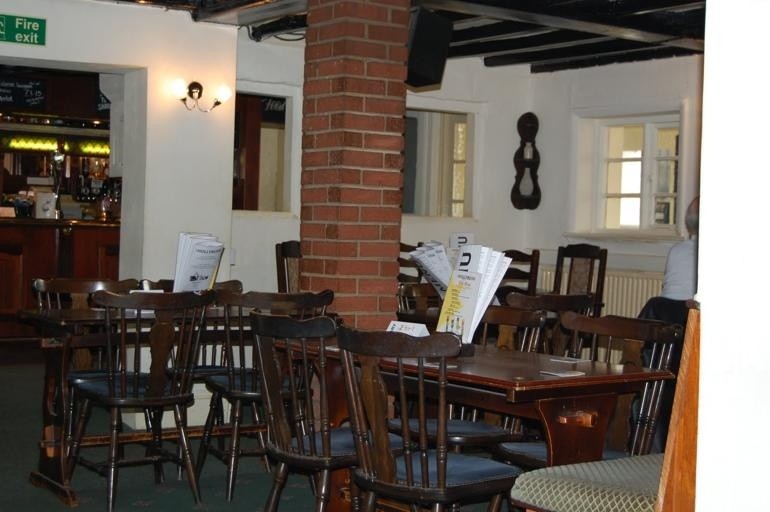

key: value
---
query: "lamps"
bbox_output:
[170,80,232,113]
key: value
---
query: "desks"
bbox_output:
[275,332,676,465]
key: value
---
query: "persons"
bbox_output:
[661,196,699,301]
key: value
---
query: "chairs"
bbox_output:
[642,295,695,422]
[248,309,413,512]
[382,311,547,451]
[335,324,524,512]
[494,311,684,465]
[273,240,608,374]
[18,273,335,510]
[507,307,700,510]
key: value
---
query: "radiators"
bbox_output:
[526,269,672,364]
